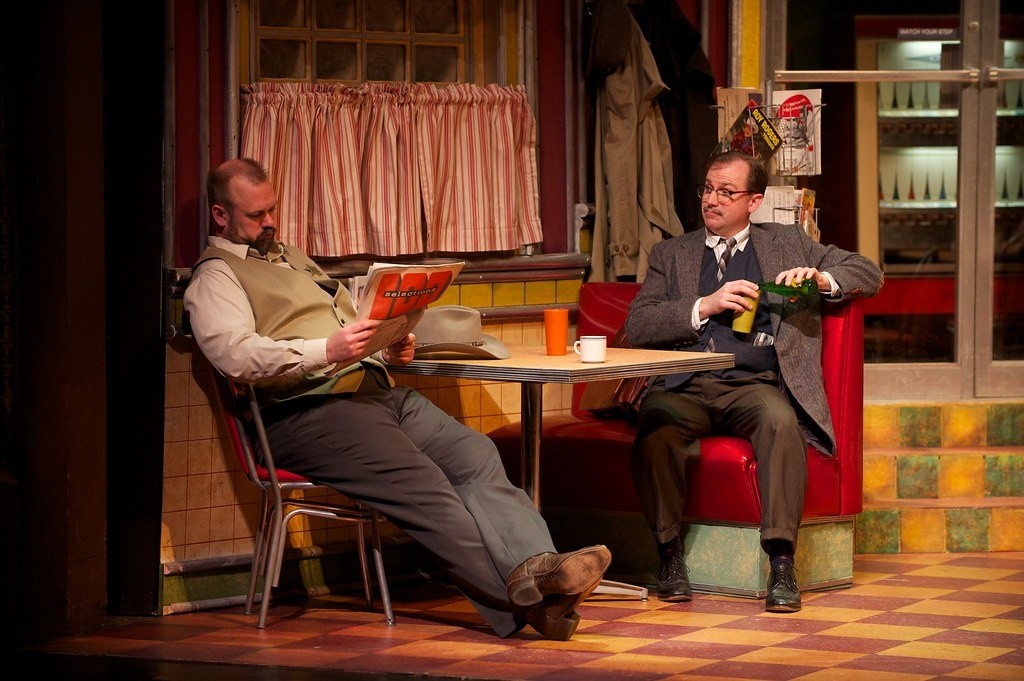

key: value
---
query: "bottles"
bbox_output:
[756,278,817,297]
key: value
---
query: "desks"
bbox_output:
[386,348,736,591]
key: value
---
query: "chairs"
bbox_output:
[183,312,396,628]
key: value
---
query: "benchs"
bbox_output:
[485,283,866,600]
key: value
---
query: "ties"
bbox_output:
[702,238,737,355]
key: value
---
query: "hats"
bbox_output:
[410,306,510,359]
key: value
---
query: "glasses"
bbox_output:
[696,183,759,204]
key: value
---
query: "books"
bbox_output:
[771,89,823,176]
[711,88,784,167]
[749,186,821,248]
[940,43,962,110]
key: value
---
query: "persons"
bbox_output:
[624,152,881,611]
[184,157,613,642]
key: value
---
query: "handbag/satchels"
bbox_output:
[579,322,648,418]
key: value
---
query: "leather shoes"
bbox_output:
[506,545,612,606]
[657,552,693,601]
[526,575,603,641]
[765,560,802,612]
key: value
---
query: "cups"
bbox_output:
[732,290,762,333]
[544,309,569,356]
[574,335,607,362]
